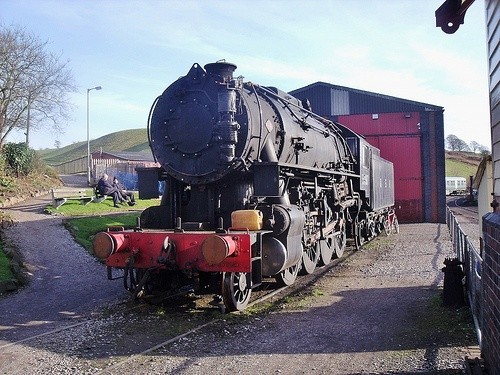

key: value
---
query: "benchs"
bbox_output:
[52,187,113,210]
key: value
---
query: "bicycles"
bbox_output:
[384,206,402,235]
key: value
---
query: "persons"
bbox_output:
[98,174,129,208]
[113,176,137,207]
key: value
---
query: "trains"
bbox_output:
[93,59,400,310]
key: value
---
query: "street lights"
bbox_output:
[84,84,103,186]
